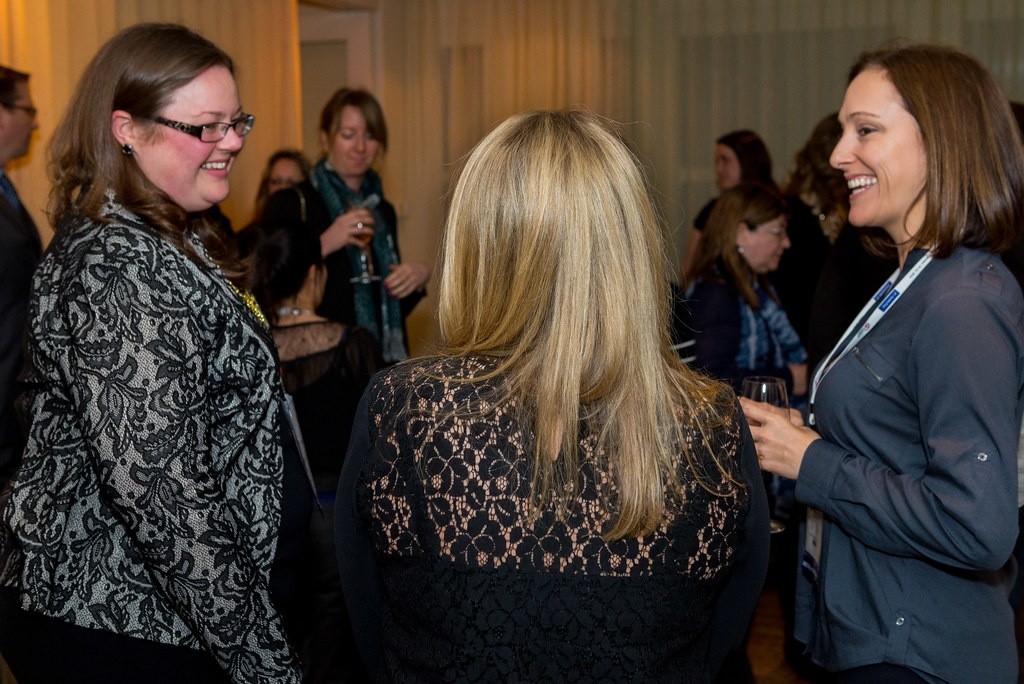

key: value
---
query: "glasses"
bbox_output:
[12,103,36,118]
[146,112,255,143]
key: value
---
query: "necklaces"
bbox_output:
[221,274,268,325]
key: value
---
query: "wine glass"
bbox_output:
[741,376,790,533]
[346,206,380,282]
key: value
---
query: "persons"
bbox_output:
[0,65,48,381]
[233,85,433,514]
[733,44,1024,684]
[0,23,364,684]
[671,107,900,661]
[329,107,772,684]
